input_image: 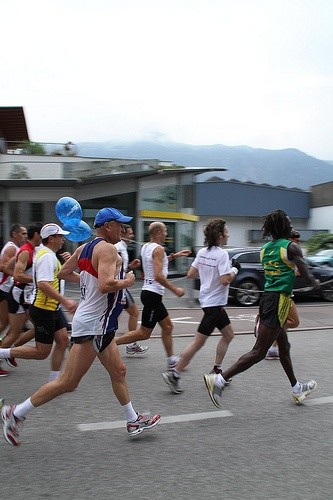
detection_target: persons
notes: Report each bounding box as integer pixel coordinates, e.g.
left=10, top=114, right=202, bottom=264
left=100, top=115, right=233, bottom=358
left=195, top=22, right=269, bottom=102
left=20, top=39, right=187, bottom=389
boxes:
left=113, top=221, right=149, bottom=356
left=162, top=218, right=241, bottom=394
left=203, top=212, right=320, bottom=408
left=0, top=223, right=72, bottom=378
left=0, top=223, right=79, bottom=393
left=254, top=229, right=302, bottom=359
left=113, top=221, right=192, bottom=369
left=0, top=207, right=162, bottom=448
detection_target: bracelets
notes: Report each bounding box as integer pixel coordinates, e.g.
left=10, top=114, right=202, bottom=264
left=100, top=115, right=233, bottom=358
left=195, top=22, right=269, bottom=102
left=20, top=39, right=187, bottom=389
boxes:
left=232, top=267, right=239, bottom=275
left=171, top=253, right=175, bottom=260
left=128, top=266, right=133, bottom=270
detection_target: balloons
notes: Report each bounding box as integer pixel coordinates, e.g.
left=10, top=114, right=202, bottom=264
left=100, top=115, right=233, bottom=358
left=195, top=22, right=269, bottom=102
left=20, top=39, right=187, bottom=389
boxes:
left=62, top=218, right=94, bottom=243
left=55, top=197, right=82, bottom=226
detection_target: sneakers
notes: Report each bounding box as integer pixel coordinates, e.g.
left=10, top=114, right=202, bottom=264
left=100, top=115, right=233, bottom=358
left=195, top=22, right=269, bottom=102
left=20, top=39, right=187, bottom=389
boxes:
left=162, top=370, right=183, bottom=394
left=127, top=412, right=161, bottom=437
left=168, top=358, right=178, bottom=370
left=0, top=370, right=10, bottom=377
left=292, top=379, right=317, bottom=403
left=5, top=357, right=17, bottom=367
left=265, top=347, right=281, bottom=360
left=1, top=404, right=26, bottom=446
left=204, top=373, right=224, bottom=408
left=126, top=342, right=149, bottom=356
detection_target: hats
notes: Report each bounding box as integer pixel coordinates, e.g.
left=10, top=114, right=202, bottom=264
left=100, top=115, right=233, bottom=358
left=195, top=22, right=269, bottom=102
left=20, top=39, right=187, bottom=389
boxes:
left=93, top=208, right=134, bottom=227
left=40, top=223, right=70, bottom=239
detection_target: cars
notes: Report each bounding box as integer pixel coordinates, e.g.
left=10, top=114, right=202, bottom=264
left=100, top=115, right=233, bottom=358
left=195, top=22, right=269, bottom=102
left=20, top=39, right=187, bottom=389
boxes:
left=193, top=246, right=333, bottom=307
